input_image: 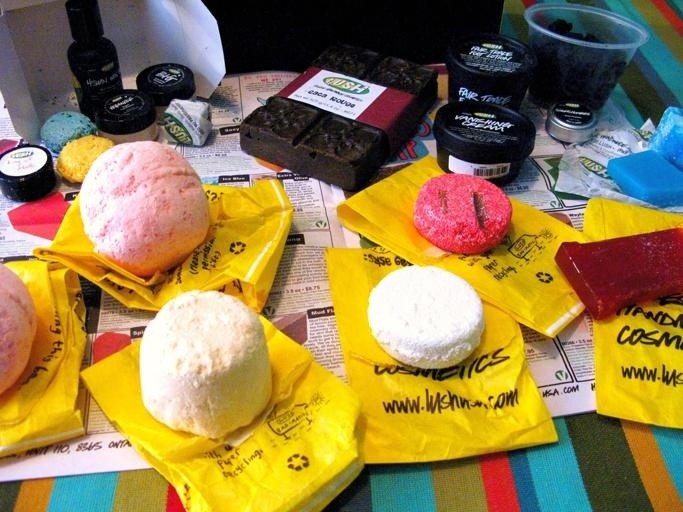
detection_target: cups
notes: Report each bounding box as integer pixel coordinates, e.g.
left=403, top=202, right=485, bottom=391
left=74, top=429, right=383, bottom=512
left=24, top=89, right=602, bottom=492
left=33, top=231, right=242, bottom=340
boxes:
left=523, top=2, right=652, bottom=112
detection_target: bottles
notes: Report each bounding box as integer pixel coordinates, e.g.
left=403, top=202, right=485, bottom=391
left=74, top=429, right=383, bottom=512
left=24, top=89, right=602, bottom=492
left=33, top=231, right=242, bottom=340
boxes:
left=66, top=1, right=123, bottom=114
